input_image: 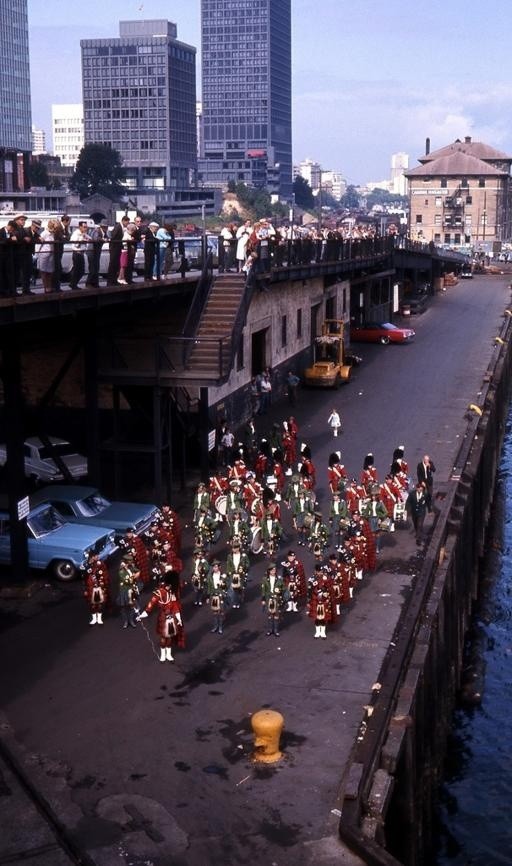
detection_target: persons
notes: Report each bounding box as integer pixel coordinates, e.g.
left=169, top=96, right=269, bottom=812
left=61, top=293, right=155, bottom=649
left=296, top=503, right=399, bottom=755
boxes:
left=218, top=219, right=399, bottom=274
left=190, top=442, right=435, bottom=637
left=82, top=505, right=184, bottom=666
left=1, top=209, right=177, bottom=298
left=404, top=227, right=511, bottom=265
left=327, top=409, right=342, bottom=437
left=247, top=368, right=303, bottom=462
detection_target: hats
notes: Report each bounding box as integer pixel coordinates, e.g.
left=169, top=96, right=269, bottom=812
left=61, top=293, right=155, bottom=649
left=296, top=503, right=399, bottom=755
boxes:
left=263, top=488, right=276, bottom=506
left=363, top=453, right=373, bottom=469
left=301, top=443, right=311, bottom=459
left=330, top=451, right=342, bottom=466
left=391, top=449, right=404, bottom=474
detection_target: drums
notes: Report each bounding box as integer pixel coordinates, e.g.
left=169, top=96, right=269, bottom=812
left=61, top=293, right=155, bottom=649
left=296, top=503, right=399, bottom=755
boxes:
left=249, top=496, right=259, bottom=514
left=393, top=502, right=408, bottom=522
left=380, top=518, right=392, bottom=532
left=215, top=494, right=227, bottom=515
left=249, top=525, right=264, bottom=555
left=303, top=514, right=315, bottom=529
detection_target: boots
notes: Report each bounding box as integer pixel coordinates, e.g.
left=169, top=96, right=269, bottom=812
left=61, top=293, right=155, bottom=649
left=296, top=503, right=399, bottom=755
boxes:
left=90, top=613, right=102, bottom=625
left=211, top=624, right=223, bottom=633
left=356, top=568, right=362, bottom=579
left=123, top=618, right=137, bottom=628
left=314, top=625, right=326, bottom=639
left=349, top=587, right=354, bottom=598
left=285, top=601, right=298, bottom=612
left=336, top=604, right=340, bottom=615
left=160, top=647, right=175, bottom=663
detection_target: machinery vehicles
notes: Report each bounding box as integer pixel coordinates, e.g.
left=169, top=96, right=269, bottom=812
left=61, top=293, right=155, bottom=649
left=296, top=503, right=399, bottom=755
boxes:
left=303, top=316, right=352, bottom=391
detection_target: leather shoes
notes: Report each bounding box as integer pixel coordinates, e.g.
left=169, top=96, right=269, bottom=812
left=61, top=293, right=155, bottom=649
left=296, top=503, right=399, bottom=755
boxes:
left=266, top=631, right=280, bottom=637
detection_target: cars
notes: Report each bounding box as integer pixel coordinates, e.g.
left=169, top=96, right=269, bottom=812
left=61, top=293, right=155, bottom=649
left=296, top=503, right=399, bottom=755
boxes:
left=3, top=434, right=163, bottom=579
left=346, top=321, right=416, bottom=348
left=1, top=210, right=223, bottom=277
